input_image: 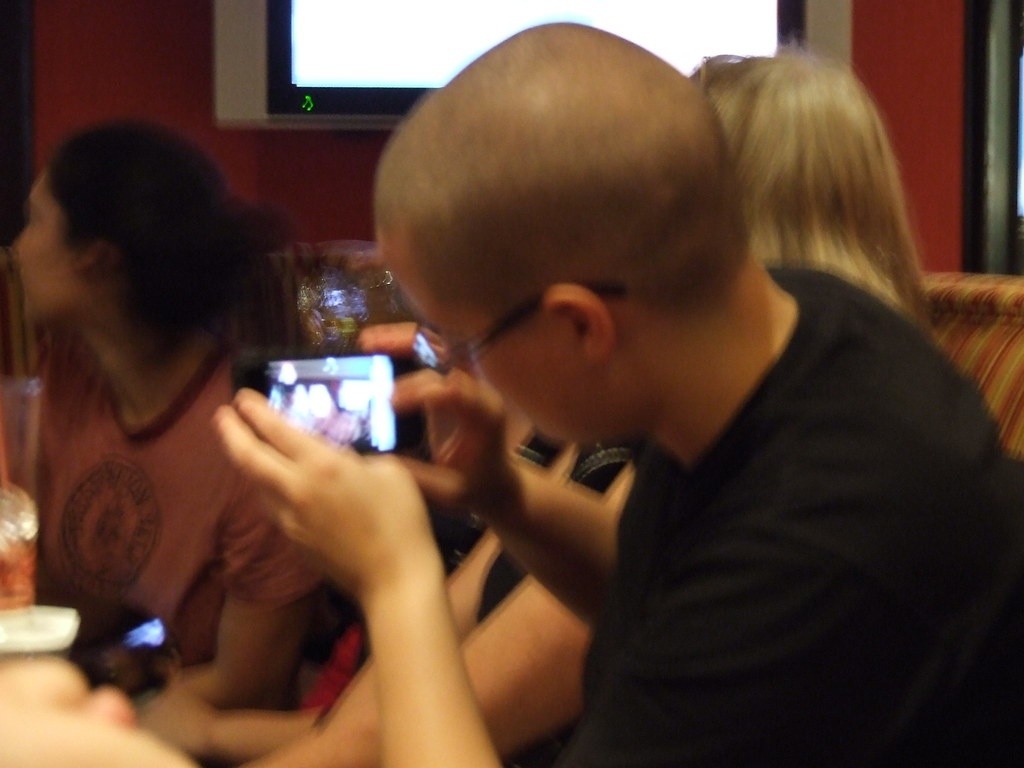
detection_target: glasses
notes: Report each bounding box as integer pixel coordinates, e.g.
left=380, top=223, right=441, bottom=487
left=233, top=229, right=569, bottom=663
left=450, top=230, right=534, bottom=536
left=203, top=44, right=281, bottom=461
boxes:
left=699, top=54, right=748, bottom=96
left=412, top=278, right=628, bottom=375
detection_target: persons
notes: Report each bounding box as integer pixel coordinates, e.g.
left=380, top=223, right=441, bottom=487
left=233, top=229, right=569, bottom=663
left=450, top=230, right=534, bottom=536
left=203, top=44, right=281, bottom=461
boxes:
left=214, top=21, right=1024, bottom=768
left=1, top=47, right=928, bottom=768
left=305, top=383, right=357, bottom=442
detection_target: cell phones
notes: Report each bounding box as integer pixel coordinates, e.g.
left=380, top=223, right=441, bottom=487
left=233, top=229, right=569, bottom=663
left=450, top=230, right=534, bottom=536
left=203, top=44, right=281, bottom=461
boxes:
left=235, top=345, right=425, bottom=460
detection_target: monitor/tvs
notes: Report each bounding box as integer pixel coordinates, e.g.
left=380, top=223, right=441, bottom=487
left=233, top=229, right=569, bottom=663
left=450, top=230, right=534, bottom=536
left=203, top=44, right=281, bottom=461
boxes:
left=211, top=0, right=853, bottom=133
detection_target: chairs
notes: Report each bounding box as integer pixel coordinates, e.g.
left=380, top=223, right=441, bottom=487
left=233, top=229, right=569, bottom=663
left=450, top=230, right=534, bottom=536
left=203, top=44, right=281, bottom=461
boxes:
left=922, top=272, right=1024, bottom=459
